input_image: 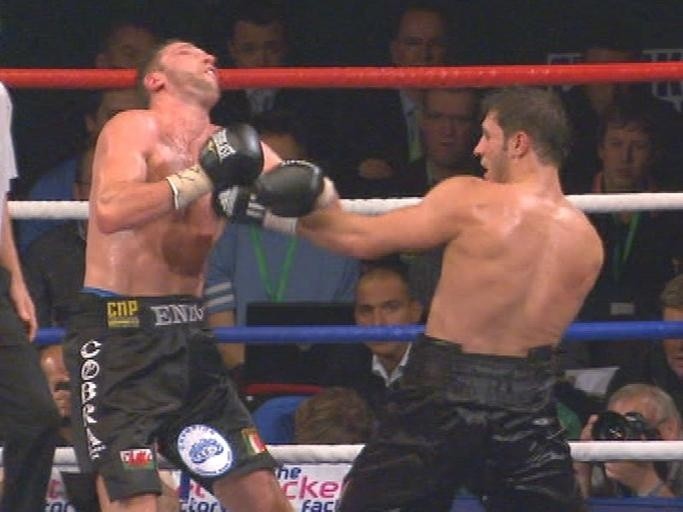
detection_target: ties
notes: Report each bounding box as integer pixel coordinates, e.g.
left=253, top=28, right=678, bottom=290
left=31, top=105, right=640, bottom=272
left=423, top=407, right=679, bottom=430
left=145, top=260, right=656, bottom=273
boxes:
left=408, top=105, right=422, bottom=166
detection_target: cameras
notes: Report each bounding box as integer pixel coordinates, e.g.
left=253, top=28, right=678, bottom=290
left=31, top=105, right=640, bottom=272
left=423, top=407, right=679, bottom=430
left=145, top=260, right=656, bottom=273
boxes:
left=592, top=411, right=668, bottom=477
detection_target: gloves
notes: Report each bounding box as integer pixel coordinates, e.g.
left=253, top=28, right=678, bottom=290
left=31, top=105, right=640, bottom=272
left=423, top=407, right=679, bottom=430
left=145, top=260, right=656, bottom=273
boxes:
left=199, top=123, right=263, bottom=190
left=212, top=184, right=270, bottom=226
left=257, top=159, right=323, bottom=218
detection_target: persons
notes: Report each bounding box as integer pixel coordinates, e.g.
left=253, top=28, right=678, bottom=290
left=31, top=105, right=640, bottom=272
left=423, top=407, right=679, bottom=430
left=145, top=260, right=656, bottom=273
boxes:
left=1, top=2, right=683, bottom=511
